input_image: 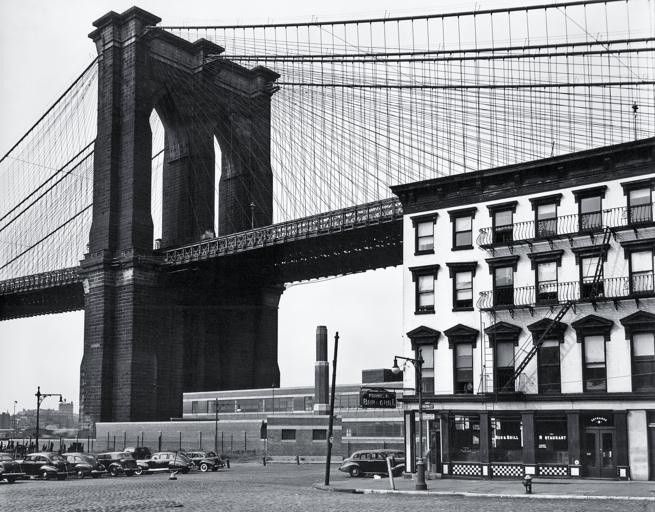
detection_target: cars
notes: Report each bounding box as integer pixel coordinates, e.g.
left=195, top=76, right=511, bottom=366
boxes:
left=0, top=447, right=225, bottom=484
left=338, top=449, right=406, bottom=478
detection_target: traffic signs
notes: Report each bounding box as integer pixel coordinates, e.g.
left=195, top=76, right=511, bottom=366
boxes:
left=359, top=391, right=396, bottom=408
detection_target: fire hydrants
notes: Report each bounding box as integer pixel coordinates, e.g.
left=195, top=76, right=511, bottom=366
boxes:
left=522, top=475, right=532, bottom=494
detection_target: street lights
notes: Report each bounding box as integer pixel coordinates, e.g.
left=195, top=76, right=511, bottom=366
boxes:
left=391, top=350, right=427, bottom=490
left=213, top=396, right=242, bottom=452
left=35, top=386, right=63, bottom=452
left=249, top=202, right=256, bottom=228
left=631, top=101, right=638, bottom=141
